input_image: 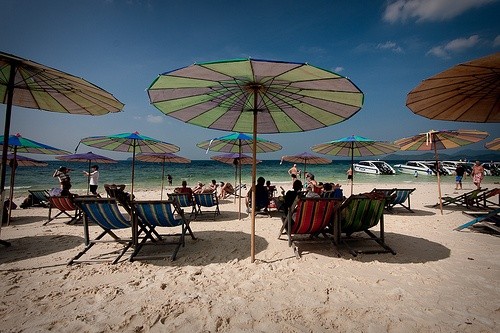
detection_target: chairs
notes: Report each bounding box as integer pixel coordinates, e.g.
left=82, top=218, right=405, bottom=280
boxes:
left=75, top=194, right=102, bottom=223
left=167, top=192, right=198, bottom=220
left=322, top=193, right=398, bottom=257
left=128, top=198, right=198, bottom=262
left=194, top=193, right=221, bottom=221
left=369, top=188, right=396, bottom=214
left=434, top=187, right=488, bottom=209
left=42, top=196, right=83, bottom=225
left=264, top=185, right=277, bottom=200
left=277, top=196, right=346, bottom=259
left=66, top=197, right=146, bottom=265
left=385, top=188, right=416, bottom=214
left=453, top=209, right=500, bottom=233
left=247, top=192, right=271, bottom=219
left=107, top=191, right=135, bottom=215
left=29, top=190, right=56, bottom=208
left=462, top=188, right=500, bottom=209
left=224, top=184, right=246, bottom=200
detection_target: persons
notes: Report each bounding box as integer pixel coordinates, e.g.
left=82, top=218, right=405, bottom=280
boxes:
left=414, top=170, right=418, bottom=178
left=219, top=181, right=234, bottom=198
left=470, top=160, right=484, bottom=189
left=200, top=179, right=217, bottom=193
left=266, top=181, right=270, bottom=186
left=287, top=163, right=299, bottom=189
left=346, top=167, right=353, bottom=184
left=106, top=183, right=126, bottom=191
left=453, top=163, right=464, bottom=189
left=489, top=160, right=500, bottom=175
left=458, top=156, right=469, bottom=162
left=308, top=185, right=343, bottom=198
left=320, top=182, right=324, bottom=185
left=280, top=180, right=304, bottom=215
left=190, top=181, right=202, bottom=195
left=429, top=159, right=445, bottom=173
left=83, top=165, right=100, bottom=195
left=166, top=173, right=173, bottom=185
left=245, top=177, right=270, bottom=213
left=173, top=179, right=193, bottom=194
left=52, top=166, right=73, bottom=193
left=306, top=172, right=317, bottom=187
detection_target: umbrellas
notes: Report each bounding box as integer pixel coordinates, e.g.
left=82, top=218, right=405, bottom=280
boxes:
left=0, top=134, right=72, bottom=227
left=405, top=53, right=500, bottom=123
left=196, top=132, right=282, bottom=219
left=394, top=128, right=489, bottom=215
left=309, top=134, right=399, bottom=196
left=135, top=151, right=191, bottom=201
left=144, top=56, right=364, bottom=264
left=1, top=153, right=49, bottom=198
left=210, top=152, right=262, bottom=204
left=483, top=137, right=500, bottom=151
left=0, top=51, right=125, bottom=192
left=280, top=151, right=331, bottom=183
left=80, top=131, right=180, bottom=201
left=56, top=152, right=117, bottom=195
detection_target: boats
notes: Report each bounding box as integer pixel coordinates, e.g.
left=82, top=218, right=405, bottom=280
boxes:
left=443, top=160, right=476, bottom=175
left=479, top=161, right=500, bottom=175
left=353, top=160, right=397, bottom=174
left=394, top=160, right=450, bottom=175
